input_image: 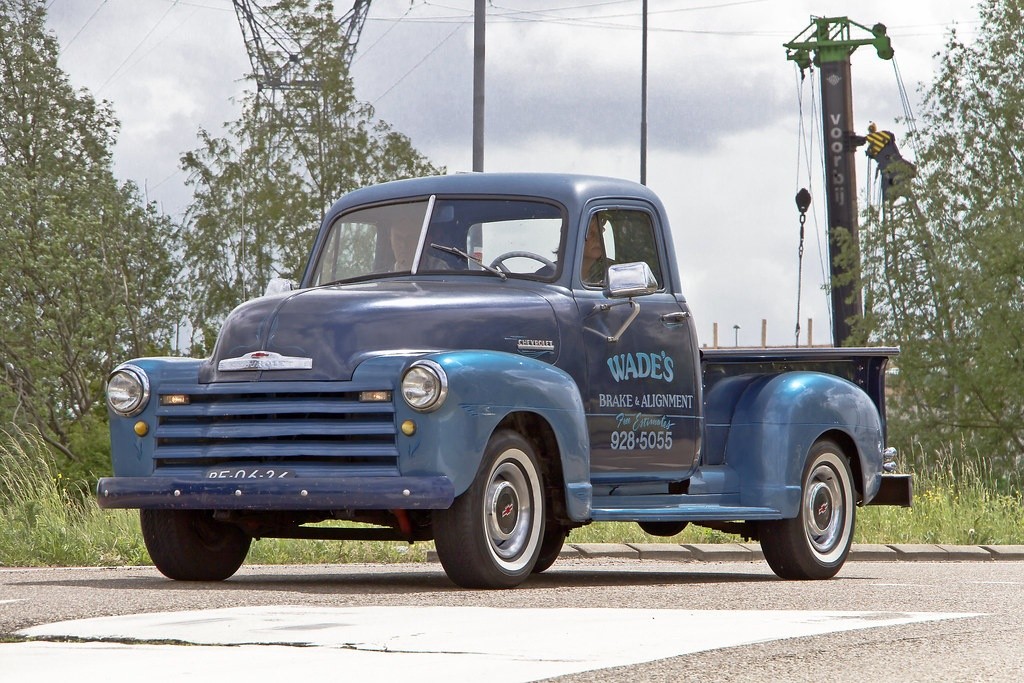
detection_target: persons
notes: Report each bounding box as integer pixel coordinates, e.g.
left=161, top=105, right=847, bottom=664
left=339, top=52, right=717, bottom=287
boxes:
left=530, top=207, right=625, bottom=289
left=371, top=207, right=453, bottom=282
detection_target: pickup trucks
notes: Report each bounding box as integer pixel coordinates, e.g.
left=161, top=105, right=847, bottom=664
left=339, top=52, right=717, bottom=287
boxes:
left=96, top=172, right=916, bottom=589
